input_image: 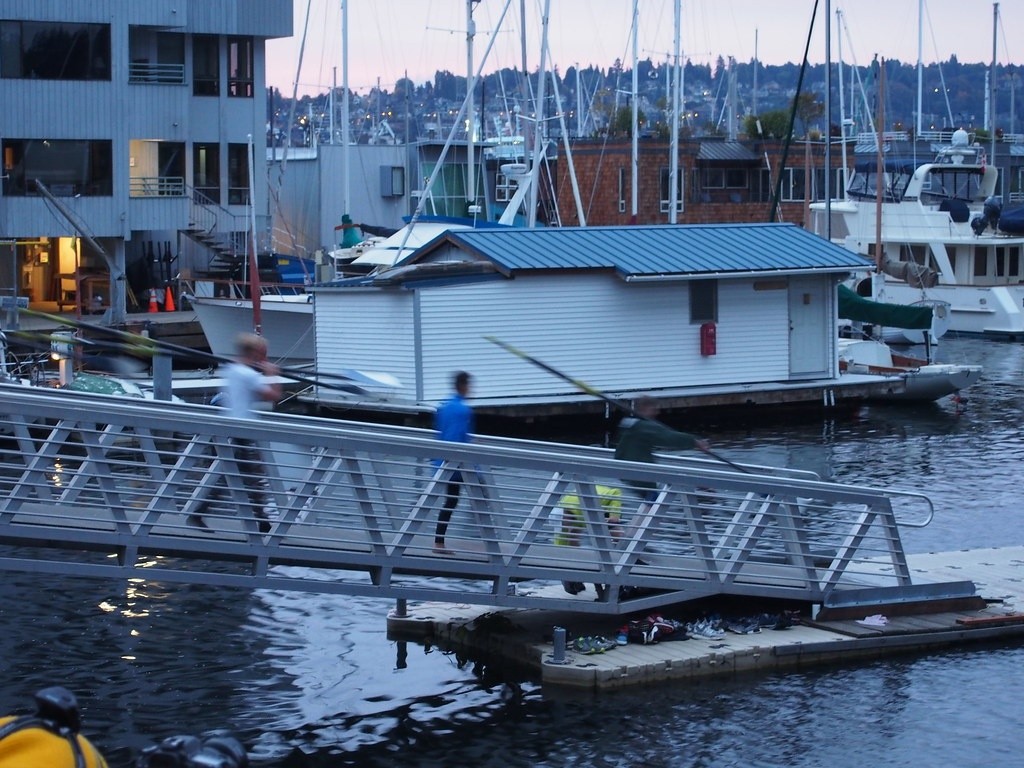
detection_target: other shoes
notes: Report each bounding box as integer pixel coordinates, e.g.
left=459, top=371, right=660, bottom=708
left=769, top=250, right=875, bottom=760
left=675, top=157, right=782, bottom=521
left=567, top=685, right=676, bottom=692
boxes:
left=855, top=614, right=888, bottom=626
left=186, top=516, right=215, bottom=533
left=543, top=632, right=574, bottom=649
left=258, top=523, right=271, bottom=532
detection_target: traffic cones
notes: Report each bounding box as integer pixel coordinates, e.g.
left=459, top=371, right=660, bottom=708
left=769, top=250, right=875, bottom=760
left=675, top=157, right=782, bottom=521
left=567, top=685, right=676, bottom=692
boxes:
left=165, top=285, right=178, bottom=313
left=148, top=290, right=161, bottom=312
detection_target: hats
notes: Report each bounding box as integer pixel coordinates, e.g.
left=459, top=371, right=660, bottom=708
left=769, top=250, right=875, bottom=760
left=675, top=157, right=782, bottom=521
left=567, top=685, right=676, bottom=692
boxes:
left=549, top=507, right=564, bottom=532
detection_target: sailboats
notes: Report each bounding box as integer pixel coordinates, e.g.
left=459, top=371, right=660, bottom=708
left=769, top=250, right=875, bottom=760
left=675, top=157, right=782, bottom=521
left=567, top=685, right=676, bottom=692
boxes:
left=186, top=134, right=314, bottom=377
left=772, top=0, right=1024, bottom=402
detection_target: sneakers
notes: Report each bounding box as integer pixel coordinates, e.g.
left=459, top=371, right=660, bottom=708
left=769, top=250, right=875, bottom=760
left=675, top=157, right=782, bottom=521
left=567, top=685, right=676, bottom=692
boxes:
left=593, top=635, right=616, bottom=650
left=572, top=637, right=595, bottom=654
left=616, top=611, right=799, bottom=646
left=585, top=636, right=605, bottom=654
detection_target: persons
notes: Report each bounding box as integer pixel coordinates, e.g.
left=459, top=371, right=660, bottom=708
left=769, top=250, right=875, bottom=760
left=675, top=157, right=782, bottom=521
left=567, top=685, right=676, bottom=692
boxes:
left=549, top=396, right=710, bottom=546
left=423, top=372, right=489, bottom=557
left=189, top=332, right=278, bottom=533
